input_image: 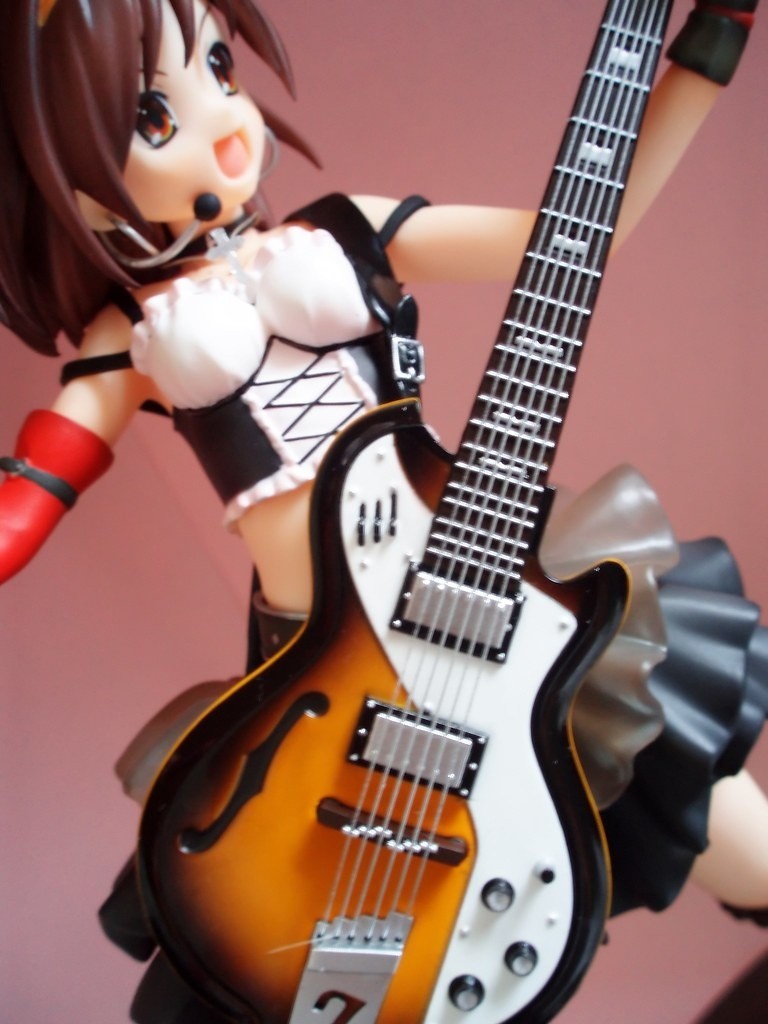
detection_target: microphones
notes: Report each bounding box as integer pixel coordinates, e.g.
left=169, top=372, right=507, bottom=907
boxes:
left=95, top=192, right=221, bottom=269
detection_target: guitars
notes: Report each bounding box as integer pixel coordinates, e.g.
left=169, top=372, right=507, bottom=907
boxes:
left=136, top=0, right=675, bottom=1024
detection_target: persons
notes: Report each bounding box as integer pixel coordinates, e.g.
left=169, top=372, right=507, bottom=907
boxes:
left=0, top=0, right=768, bottom=1024
